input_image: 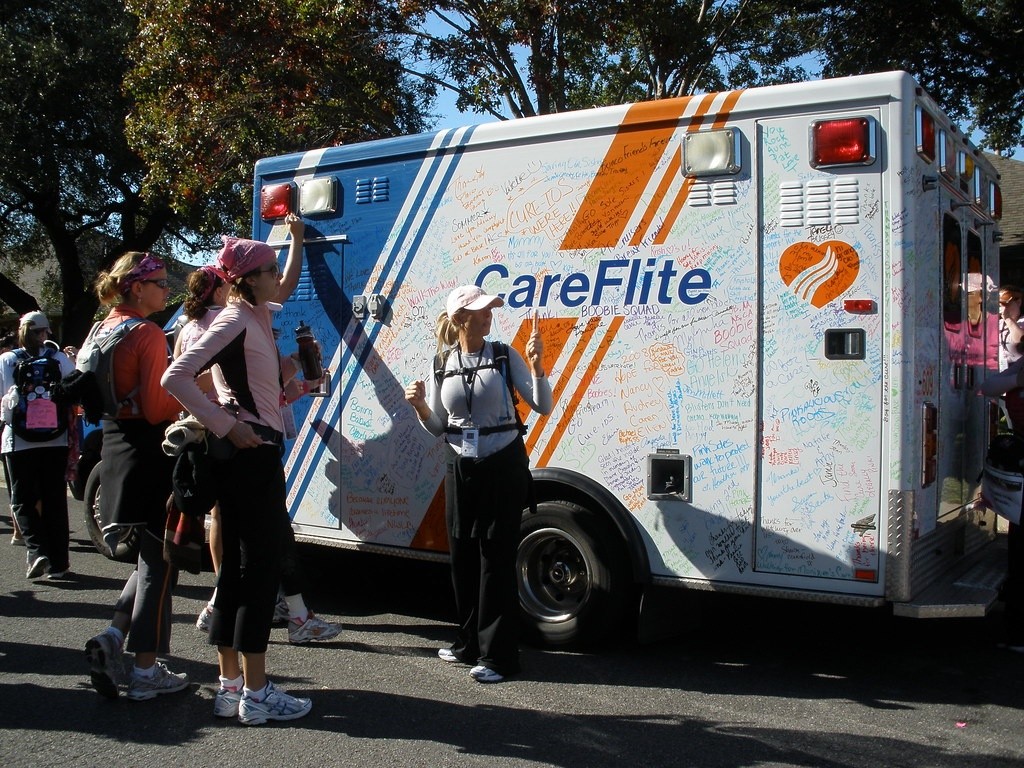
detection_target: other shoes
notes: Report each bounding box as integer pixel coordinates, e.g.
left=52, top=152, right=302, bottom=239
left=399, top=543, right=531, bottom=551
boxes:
left=11, top=536, right=26, bottom=545
left=996, top=630, right=1024, bottom=652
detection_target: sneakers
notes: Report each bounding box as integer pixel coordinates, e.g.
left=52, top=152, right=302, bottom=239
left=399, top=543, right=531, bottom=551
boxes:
left=288, top=610, right=341, bottom=643
left=214, top=676, right=245, bottom=717
left=25, top=551, right=48, bottom=580
left=437, top=648, right=466, bottom=663
left=270, top=595, right=290, bottom=622
left=238, top=679, right=312, bottom=726
left=126, top=662, right=189, bottom=701
left=47, top=569, right=80, bottom=583
left=469, top=665, right=504, bottom=682
left=195, top=607, right=212, bottom=633
left=85, top=630, right=123, bottom=698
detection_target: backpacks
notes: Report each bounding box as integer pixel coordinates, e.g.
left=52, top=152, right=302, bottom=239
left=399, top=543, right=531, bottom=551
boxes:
left=980, top=434, right=1023, bottom=526
left=9, top=349, right=71, bottom=443
left=75, top=319, right=153, bottom=427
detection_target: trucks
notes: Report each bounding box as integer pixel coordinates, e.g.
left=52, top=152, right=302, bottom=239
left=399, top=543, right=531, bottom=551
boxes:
left=65, top=68, right=1024, bottom=661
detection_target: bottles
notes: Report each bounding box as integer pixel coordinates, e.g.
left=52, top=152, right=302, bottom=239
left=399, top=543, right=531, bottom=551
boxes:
left=295, top=320, right=322, bottom=380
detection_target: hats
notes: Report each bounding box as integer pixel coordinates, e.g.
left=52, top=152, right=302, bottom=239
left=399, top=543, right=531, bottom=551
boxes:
left=20, top=310, right=52, bottom=334
left=446, top=285, right=504, bottom=321
left=998, top=284, right=1022, bottom=303
left=959, top=272, right=997, bottom=293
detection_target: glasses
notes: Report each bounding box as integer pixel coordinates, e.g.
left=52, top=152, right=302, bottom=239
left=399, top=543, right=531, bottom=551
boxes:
left=126, top=279, right=169, bottom=289
left=244, top=262, right=281, bottom=279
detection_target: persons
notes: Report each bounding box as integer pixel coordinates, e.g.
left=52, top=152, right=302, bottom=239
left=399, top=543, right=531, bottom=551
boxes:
left=75, top=252, right=188, bottom=700
left=0, top=311, right=79, bottom=579
left=160, top=213, right=342, bottom=726
left=997, top=281, right=1024, bottom=463
left=949, top=272, right=997, bottom=401
left=405, top=285, right=552, bottom=684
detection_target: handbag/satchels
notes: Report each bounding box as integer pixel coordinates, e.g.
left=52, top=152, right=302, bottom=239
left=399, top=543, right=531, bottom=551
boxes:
left=171, top=430, right=229, bottom=517
